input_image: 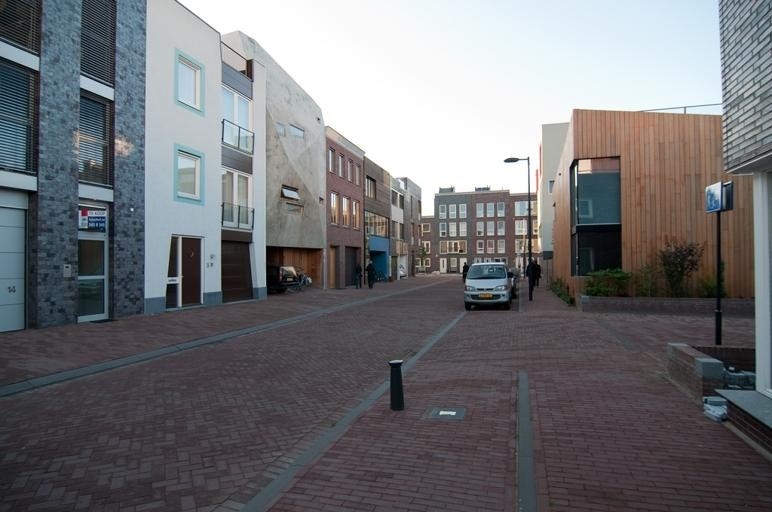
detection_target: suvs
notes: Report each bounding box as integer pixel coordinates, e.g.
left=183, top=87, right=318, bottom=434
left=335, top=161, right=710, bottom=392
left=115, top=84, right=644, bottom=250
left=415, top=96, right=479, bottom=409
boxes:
left=267, top=265, right=300, bottom=294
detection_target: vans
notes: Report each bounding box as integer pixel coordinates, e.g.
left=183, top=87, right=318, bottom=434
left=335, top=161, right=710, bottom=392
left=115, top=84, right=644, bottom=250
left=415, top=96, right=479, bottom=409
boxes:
left=463, top=261, right=515, bottom=310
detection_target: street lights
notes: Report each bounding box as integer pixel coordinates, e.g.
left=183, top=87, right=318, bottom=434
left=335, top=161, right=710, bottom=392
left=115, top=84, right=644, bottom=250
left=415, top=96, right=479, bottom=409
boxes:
left=503, top=158, right=531, bottom=268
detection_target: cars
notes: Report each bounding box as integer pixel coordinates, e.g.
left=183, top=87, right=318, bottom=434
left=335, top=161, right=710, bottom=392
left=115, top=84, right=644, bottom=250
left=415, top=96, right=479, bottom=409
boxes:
left=399, top=264, right=408, bottom=277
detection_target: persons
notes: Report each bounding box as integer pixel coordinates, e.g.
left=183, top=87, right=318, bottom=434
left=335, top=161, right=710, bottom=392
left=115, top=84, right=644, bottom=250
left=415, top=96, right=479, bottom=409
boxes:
left=534, top=260, right=541, bottom=286
left=463, top=262, right=469, bottom=282
left=525, top=262, right=537, bottom=301
left=354, top=261, right=365, bottom=291
left=364, top=259, right=376, bottom=288
